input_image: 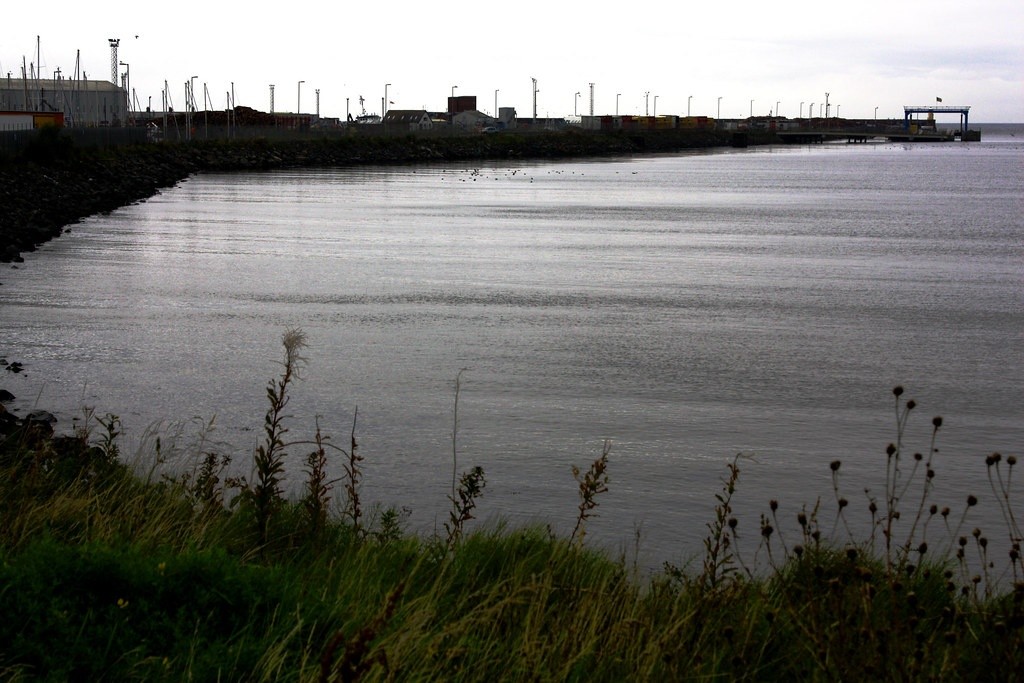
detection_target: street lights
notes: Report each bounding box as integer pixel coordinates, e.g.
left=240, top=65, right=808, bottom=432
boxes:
left=532, top=77, right=539, bottom=124
left=190, top=76, right=199, bottom=128
left=588, top=82, right=595, bottom=116
left=347, top=98, right=349, bottom=117
left=269, top=85, right=275, bottom=116
left=315, top=89, right=320, bottom=115
left=298, top=81, right=305, bottom=115
left=616, top=93, right=622, bottom=115
left=750, top=99, right=755, bottom=117
left=717, top=97, right=722, bottom=125
left=688, top=95, right=693, bottom=116
left=119, top=61, right=130, bottom=111
left=385, top=84, right=391, bottom=114
left=494, top=89, right=500, bottom=118
left=52, top=71, right=61, bottom=110
left=574, top=91, right=580, bottom=117
left=775, top=102, right=782, bottom=117
left=798, top=92, right=841, bottom=128
left=452, top=86, right=457, bottom=117
left=654, top=95, right=659, bottom=117
left=107, top=38, right=120, bottom=119
left=874, top=106, right=879, bottom=120
